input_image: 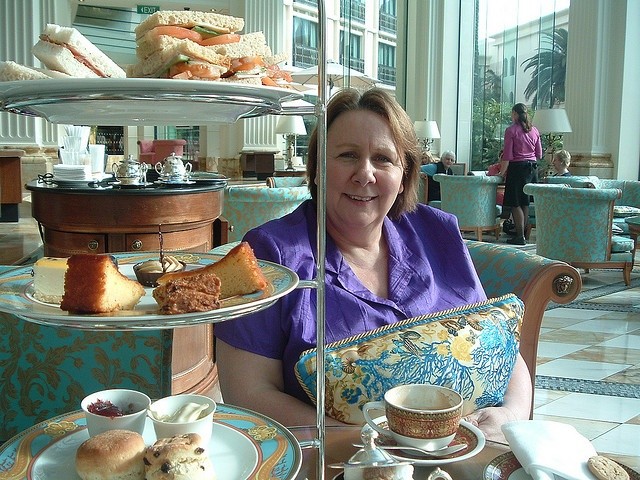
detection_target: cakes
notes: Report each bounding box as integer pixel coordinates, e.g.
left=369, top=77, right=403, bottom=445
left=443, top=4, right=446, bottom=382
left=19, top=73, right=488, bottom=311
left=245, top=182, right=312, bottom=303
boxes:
left=33, top=257, right=70, bottom=305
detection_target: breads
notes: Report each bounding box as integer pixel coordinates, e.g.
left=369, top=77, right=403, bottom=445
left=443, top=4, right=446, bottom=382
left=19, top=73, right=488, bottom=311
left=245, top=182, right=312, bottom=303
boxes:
left=0, top=60, right=52, bottom=82
left=152, top=273, right=221, bottom=315
left=156, top=241, right=269, bottom=300
left=22, top=65, right=73, bottom=78
left=144, top=433, right=209, bottom=480
left=60, top=252, right=146, bottom=314
left=75, top=429, right=144, bottom=480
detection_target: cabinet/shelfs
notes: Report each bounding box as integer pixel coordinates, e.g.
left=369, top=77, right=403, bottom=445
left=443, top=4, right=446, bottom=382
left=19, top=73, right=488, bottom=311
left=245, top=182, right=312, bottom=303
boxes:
left=239, top=150, right=256, bottom=178
left=0, top=149, right=26, bottom=222
left=254, top=150, right=280, bottom=181
left=274, top=169, right=309, bottom=176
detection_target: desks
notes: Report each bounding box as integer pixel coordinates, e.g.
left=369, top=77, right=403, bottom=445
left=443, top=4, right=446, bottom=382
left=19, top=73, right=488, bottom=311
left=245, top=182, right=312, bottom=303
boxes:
left=625, top=217, right=640, bottom=271
left=24, top=169, right=235, bottom=398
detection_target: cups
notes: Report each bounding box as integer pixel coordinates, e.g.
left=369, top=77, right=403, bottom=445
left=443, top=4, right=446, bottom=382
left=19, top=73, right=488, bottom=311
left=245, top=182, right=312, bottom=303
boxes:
left=81, top=389, right=152, bottom=438
left=362, top=384, right=463, bottom=454
left=147, top=394, right=217, bottom=444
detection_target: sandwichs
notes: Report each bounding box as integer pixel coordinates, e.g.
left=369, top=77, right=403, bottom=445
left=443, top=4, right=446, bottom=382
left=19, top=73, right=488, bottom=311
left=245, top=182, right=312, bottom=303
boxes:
left=31, top=23, right=126, bottom=78
left=126, top=10, right=294, bottom=89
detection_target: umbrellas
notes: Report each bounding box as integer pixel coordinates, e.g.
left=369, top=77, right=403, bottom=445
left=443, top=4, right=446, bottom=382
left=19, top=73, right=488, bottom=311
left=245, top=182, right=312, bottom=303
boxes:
left=289, top=59, right=381, bottom=99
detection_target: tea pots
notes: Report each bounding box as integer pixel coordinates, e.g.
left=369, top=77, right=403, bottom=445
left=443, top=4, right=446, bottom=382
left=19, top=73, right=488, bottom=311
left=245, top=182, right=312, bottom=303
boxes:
left=112, top=155, right=148, bottom=184
left=154, top=152, right=193, bottom=181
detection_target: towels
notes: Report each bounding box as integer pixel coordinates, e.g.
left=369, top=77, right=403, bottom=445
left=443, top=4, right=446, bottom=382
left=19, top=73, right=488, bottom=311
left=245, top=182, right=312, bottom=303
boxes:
left=501, top=422, right=599, bottom=480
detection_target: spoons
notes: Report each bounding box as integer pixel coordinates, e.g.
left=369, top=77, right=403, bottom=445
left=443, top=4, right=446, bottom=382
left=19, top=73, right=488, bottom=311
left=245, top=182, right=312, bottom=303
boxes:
left=351, top=441, right=468, bottom=458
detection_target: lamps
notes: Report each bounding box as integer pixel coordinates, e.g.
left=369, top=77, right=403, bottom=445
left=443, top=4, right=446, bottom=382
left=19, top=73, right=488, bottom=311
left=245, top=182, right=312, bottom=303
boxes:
left=532, top=108, right=574, bottom=176
left=412, top=119, right=442, bottom=151
left=274, top=114, right=308, bottom=170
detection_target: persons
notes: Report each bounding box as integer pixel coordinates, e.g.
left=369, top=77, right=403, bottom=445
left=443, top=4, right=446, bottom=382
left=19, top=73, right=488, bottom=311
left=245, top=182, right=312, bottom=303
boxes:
left=420, top=151, right=434, bottom=164
left=498, top=103, right=542, bottom=245
left=552, top=150, right=572, bottom=176
left=437, top=151, right=455, bottom=175
left=487, top=150, right=507, bottom=206
left=213, top=86, right=532, bottom=446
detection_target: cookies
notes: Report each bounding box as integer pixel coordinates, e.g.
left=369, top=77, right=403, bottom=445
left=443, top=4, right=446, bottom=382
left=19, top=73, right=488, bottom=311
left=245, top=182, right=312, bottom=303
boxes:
left=588, top=455, right=630, bottom=480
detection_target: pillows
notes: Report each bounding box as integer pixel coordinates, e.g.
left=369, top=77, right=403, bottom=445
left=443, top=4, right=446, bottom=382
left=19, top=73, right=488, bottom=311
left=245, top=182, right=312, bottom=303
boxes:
left=613, top=206, right=640, bottom=216
left=294, top=293, right=524, bottom=427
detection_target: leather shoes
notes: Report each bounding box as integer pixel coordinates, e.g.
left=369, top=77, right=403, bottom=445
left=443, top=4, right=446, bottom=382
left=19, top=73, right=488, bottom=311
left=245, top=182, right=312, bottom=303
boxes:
left=506, top=236, right=526, bottom=245
left=508, top=230, right=527, bottom=236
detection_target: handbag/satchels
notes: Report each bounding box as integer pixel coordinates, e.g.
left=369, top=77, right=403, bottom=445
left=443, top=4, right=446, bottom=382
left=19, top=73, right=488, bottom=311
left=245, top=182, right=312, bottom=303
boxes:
left=503, top=218, right=515, bottom=235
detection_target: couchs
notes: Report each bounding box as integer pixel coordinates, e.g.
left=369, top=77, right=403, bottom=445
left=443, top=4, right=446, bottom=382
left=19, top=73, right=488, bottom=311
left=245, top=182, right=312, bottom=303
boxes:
left=210, top=238, right=581, bottom=421
left=574, top=179, right=640, bottom=234
left=525, top=176, right=598, bottom=240
left=220, top=183, right=312, bottom=243
left=524, top=182, right=635, bottom=288
left=137, top=139, right=187, bottom=166
left=417, top=162, right=466, bottom=209
left=1, top=263, right=171, bottom=444
left=1, top=146, right=61, bottom=187
left=433, top=174, right=503, bottom=243
left=265, top=176, right=309, bottom=188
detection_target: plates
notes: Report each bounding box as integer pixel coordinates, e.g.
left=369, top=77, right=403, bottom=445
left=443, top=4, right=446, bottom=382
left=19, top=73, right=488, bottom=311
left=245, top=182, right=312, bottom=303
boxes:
left=483, top=450, right=640, bottom=480
left=108, top=181, right=153, bottom=189
left=0, top=252, right=300, bottom=330
left=154, top=181, right=196, bottom=185
left=1, top=78, right=303, bottom=126
left=52, top=179, right=95, bottom=188
left=0, top=402, right=303, bottom=480
left=361, top=414, right=486, bottom=468
